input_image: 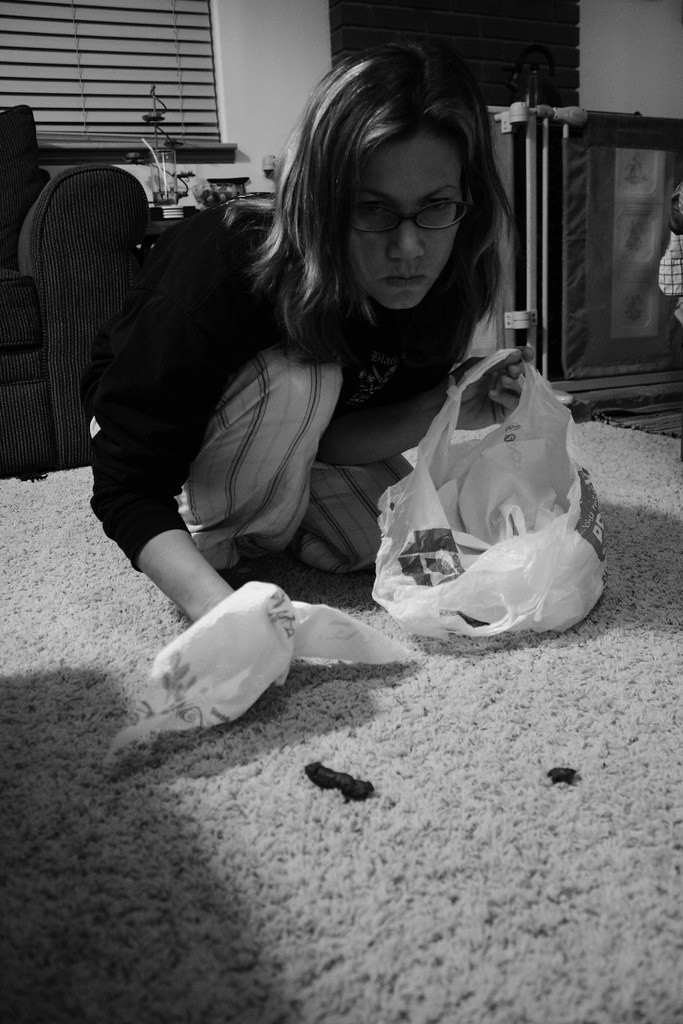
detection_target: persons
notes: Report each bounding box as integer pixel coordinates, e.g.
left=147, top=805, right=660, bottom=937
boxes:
left=659, top=183, right=683, bottom=326
left=91, top=42, right=537, bottom=625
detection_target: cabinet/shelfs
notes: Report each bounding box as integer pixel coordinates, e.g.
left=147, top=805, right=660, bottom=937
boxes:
left=502, top=113, right=683, bottom=394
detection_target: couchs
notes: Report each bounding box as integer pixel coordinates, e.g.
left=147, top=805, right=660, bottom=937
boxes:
left=0, top=169, right=165, bottom=481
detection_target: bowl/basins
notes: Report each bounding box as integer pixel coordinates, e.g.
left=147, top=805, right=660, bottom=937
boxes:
left=192, top=182, right=236, bottom=208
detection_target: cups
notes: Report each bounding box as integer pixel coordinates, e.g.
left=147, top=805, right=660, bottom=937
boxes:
left=261, top=154, right=276, bottom=176
left=149, top=149, right=177, bottom=204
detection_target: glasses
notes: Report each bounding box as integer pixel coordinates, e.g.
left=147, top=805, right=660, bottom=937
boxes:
left=350, top=182, right=473, bottom=233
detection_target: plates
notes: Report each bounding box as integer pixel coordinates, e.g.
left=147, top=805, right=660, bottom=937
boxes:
left=206, top=176, right=250, bottom=185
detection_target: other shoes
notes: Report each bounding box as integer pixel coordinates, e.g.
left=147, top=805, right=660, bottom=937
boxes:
left=218, top=560, right=272, bottom=591
left=266, top=539, right=301, bottom=572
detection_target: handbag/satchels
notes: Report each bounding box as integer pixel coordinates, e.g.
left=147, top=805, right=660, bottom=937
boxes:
left=372, top=347, right=608, bottom=639
